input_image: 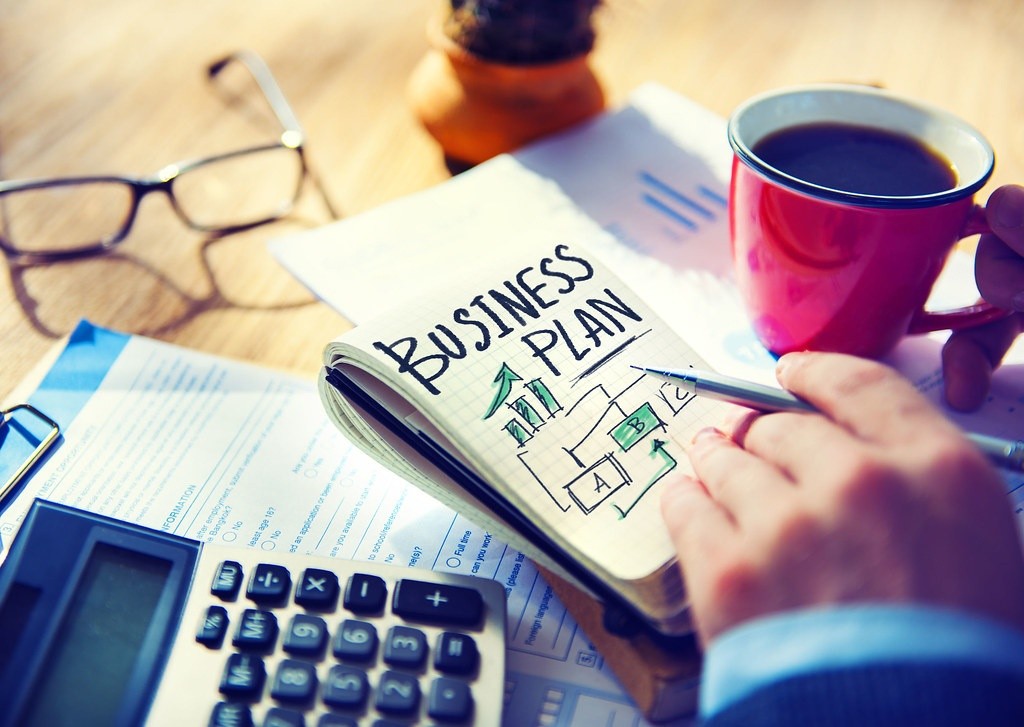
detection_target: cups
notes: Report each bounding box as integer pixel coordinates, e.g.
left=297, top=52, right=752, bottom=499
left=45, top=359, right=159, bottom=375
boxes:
left=726, top=80, right=1014, bottom=357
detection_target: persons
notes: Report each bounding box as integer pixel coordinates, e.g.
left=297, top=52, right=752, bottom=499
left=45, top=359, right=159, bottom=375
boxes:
left=663, top=185, right=1024, bottom=727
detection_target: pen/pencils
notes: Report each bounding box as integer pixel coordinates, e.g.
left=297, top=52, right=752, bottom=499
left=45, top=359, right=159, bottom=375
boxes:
left=630, top=361, right=1024, bottom=476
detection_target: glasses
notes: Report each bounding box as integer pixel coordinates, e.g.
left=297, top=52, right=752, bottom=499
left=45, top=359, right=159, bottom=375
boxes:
left=1, top=46, right=307, bottom=263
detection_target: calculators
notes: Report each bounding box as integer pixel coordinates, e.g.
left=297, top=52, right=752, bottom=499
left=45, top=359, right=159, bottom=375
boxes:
left=0, top=495, right=507, bottom=727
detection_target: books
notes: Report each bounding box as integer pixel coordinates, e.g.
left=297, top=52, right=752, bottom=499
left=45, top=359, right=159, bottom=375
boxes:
left=318, top=223, right=769, bottom=724
